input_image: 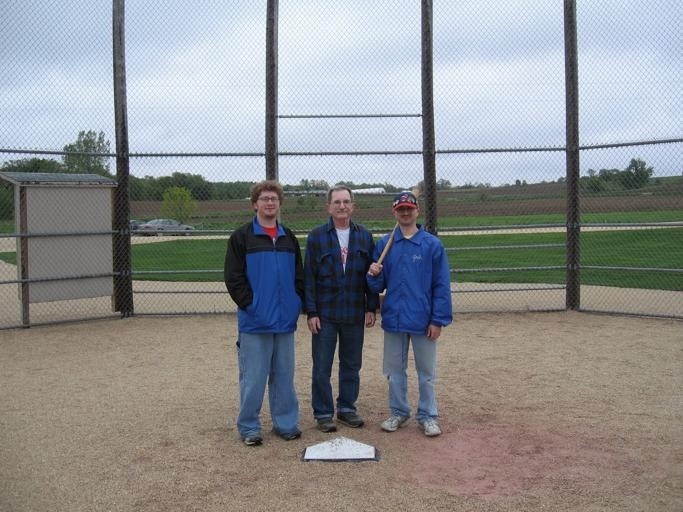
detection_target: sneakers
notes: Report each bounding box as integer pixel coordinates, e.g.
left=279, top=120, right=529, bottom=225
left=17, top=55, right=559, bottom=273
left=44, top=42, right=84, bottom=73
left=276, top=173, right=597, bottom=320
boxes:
left=242, top=435, right=262, bottom=446
left=274, top=427, right=302, bottom=440
left=335, top=410, right=365, bottom=428
left=380, top=414, right=412, bottom=432
left=417, top=417, right=442, bottom=436
left=314, top=417, right=338, bottom=433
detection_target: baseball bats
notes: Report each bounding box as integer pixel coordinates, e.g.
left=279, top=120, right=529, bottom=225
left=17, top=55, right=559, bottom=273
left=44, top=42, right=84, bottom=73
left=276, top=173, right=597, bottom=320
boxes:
left=377, top=187, right=419, bottom=264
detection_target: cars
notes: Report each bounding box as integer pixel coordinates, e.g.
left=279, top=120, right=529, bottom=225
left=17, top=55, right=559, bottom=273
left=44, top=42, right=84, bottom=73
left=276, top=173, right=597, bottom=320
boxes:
left=130, top=219, right=195, bottom=236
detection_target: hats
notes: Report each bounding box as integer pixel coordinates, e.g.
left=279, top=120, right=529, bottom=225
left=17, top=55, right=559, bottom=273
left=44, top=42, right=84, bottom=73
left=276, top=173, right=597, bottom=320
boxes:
left=392, top=190, right=419, bottom=210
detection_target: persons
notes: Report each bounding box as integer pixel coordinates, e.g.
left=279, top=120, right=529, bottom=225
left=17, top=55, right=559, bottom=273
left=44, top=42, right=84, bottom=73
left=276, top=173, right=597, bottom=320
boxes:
left=224, top=180, right=304, bottom=447
left=365, top=192, right=453, bottom=436
left=303, top=187, right=380, bottom=433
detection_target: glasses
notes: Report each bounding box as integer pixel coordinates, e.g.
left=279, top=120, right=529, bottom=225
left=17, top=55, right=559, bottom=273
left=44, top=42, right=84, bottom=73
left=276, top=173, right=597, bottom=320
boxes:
left=257, top=196, right=280, bottom=202
